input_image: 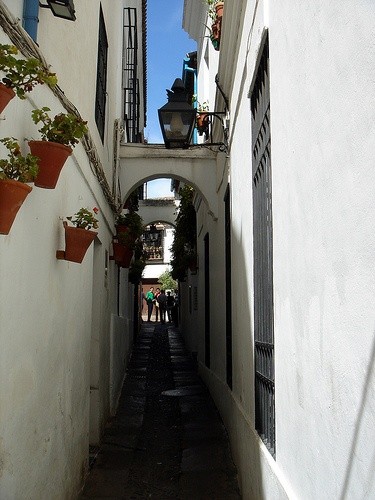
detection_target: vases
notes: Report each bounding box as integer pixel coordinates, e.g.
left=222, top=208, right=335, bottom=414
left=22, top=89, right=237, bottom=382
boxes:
left=62, top=222, right=98, bottom=265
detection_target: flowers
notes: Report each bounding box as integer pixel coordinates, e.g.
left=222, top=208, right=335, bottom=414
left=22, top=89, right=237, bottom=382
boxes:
left=67, top=206, right=100, bottom=233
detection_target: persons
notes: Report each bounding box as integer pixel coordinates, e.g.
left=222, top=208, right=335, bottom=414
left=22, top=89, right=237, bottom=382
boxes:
left=173, top=289, right=178, bottom=307
left=154, top=288, right=161, bottom=323
left=166, top=292, right=175, bottom=323
left=146, top=287, right=155, bottom=322
left=158, top=290, right=167, bottom=324
left=142, top=287, right=146, bottom=309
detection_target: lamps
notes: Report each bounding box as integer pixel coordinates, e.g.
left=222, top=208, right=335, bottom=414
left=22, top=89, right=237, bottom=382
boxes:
left=147, top=223, right=161, bottom=242
left=158, top=77, right=230, bottom=159
left=39, top=0, right=77, bottom=21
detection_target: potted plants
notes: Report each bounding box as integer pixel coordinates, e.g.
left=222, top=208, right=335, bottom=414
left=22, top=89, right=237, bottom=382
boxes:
left=0, top=44, right=59, bottom=114
left=112, top=212, right=145, bottom=270
left=0, top=135, right=42, bottom=235
left=28, top=106, right=90, bottom=190
left=192, top=93, right=210, bottom=130
left=201, top=0, right=224, bottom=51
